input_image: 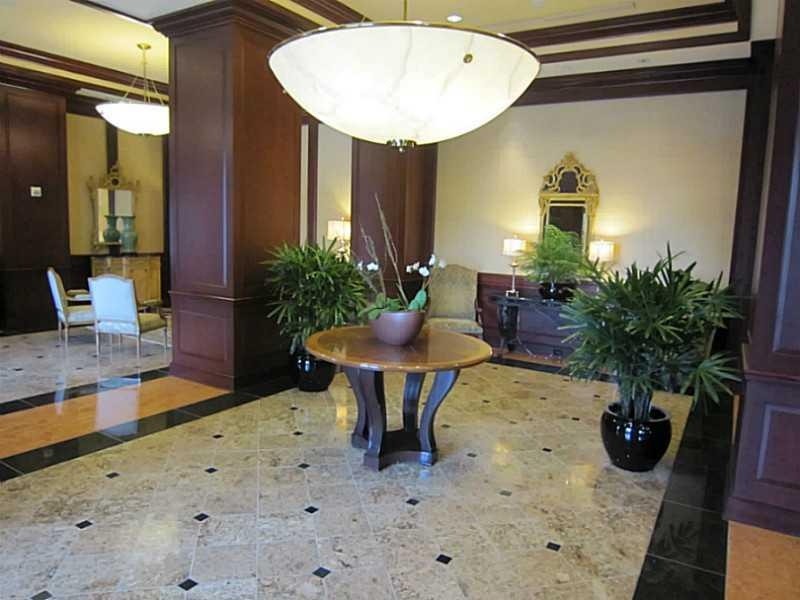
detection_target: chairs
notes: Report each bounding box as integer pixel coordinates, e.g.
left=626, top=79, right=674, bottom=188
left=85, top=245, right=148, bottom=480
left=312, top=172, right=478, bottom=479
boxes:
left=88, top=274, right=168, bottom=360
left=47, top=267, right=95, bottom=350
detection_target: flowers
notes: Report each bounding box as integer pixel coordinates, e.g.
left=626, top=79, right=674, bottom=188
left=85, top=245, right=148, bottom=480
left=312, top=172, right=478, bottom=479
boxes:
left=356, top=256, right=448, bottom=320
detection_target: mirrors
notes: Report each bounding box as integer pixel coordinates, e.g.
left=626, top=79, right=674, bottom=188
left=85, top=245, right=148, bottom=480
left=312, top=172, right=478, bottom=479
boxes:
left=300, top=107, right=352, bottom=250
left=86, top=162, right=140, bottom=252
left=539, top=153, right=599, bottom=259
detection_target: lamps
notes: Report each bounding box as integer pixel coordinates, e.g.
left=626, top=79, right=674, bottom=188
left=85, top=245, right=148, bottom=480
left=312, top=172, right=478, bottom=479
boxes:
left=327, top=218, right=351, bottom=263
left=95, top=43, right=170, bottom=137
left=588, top=240, right=615, bottom=293
left=502, top=234, right=527, bottom=298
left=266, top=0, right=542, bottom=157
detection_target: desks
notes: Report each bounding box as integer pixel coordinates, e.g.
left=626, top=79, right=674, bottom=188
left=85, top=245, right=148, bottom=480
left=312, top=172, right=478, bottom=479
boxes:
left=303, top=329, right=493, bottom=472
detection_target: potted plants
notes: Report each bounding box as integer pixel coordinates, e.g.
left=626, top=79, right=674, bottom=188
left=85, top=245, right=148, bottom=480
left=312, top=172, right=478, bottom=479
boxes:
left=558, top=240, right=759, bottom=473
left=513, top=224, right=587, bottom=302
left=257, top=234, right=372, bottom=391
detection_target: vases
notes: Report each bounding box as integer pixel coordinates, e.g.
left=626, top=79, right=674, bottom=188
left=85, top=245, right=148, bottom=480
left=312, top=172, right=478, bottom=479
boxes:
left=102, top=215, right=121, bottom=242
left=121, top=216, right=138, bottom=252
left=368, top=310, right=426, bottom=346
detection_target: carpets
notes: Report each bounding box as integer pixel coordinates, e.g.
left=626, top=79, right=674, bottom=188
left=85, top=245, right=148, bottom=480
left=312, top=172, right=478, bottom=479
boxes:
left=86, top=326, right=172, bottom=348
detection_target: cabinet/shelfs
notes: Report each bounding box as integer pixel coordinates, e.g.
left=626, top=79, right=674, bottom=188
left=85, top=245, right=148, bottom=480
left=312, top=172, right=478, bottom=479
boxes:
left=71, top=253, right=165, bottom=313
